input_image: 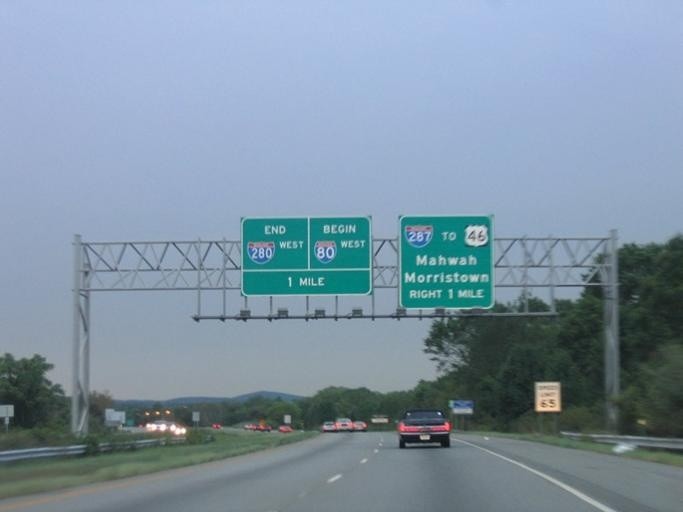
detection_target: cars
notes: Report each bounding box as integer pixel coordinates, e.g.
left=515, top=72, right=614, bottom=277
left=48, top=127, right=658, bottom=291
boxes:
left=321, top=418, right=367, bottom=433
left=144, top=419, right=187, bottom=436
left=394, top=409, right=451, bottom=448
left=211, top=423, right=220, bottom=429
left=242, top=423, right=292, bottom=433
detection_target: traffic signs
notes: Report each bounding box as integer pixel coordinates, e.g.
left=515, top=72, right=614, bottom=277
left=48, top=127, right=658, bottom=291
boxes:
left=397, top=214, right=495, bottom=309
left=239, top=214, right=372, bottom=296
left=533, top=381, right=561, bottom=413
